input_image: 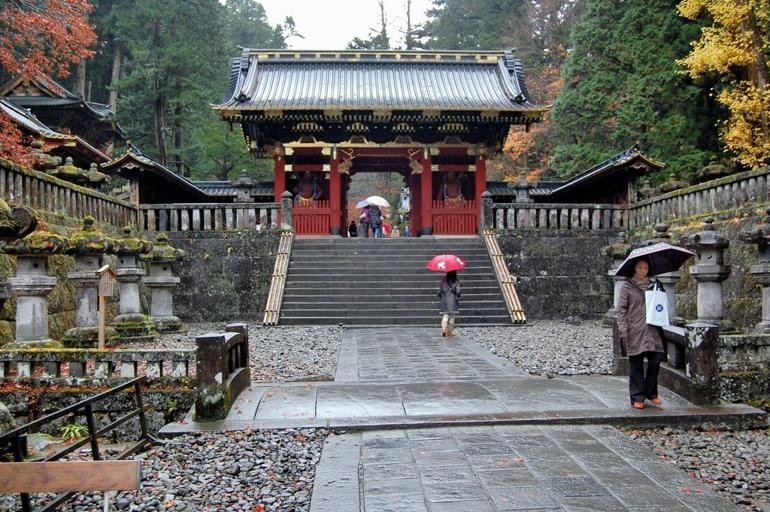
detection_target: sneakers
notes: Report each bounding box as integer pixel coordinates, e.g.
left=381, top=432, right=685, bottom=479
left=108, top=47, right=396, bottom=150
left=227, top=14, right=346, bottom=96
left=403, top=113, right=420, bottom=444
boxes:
left=631, top=398, right=660, bottom=409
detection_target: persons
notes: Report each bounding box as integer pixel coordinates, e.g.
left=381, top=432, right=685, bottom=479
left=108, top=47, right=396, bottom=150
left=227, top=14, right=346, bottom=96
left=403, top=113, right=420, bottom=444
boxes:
left=349, top=220, right=358, bottom=238
left=359, top=205, right=371, bottom=238
left=367, top=205, right=382, bottom=239
left=381, top=220, right=410, bottom=238
left=618, top=256, right=667, bottom=409
left=437, top=270, right=462, bottom=337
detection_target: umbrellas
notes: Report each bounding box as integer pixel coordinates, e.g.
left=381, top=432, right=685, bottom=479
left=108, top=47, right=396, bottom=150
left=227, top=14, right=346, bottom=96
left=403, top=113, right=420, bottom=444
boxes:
left=364, top=196, right=390, bottom=207
left=614, top=242, right=697, bottom=293
left=355, top=200, right=369, bottom=209
left=425, top=252, right=466, bottom=272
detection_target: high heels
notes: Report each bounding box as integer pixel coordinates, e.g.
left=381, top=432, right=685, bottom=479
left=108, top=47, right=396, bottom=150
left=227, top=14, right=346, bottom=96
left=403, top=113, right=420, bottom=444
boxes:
left=443, top=331, right=453, bottom=337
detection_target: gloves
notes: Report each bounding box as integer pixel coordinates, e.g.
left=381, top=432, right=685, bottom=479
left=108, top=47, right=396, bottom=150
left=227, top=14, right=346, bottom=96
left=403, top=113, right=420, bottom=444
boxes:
left=654, top=279, right=664, bottom=292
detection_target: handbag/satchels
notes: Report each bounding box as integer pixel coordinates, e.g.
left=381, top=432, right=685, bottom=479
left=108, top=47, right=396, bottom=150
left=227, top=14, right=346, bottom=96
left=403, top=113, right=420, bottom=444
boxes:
left=376, top=216, right=382, bottom=225
left=359, top=212, right=366, bottom=222
left=645, top=290, right=674, bottom=327
